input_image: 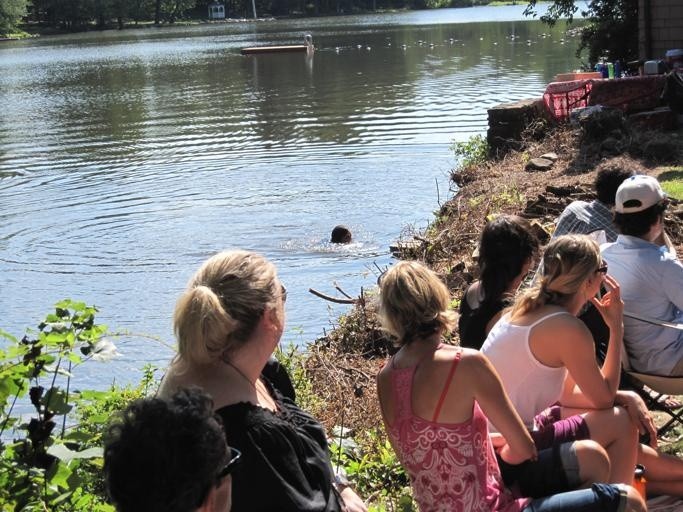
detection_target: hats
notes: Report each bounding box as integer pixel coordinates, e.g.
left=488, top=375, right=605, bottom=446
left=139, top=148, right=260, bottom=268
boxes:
left=614, top=174, right=663, bottom=214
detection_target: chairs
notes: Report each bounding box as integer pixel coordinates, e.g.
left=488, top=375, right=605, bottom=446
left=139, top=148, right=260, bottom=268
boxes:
left=596, top=287, right=683, bottom=445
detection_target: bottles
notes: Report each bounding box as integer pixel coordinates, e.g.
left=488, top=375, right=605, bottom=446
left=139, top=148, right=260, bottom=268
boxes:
left=632, top=464, right=647, bottom=506
left=595, top=61, right=621, bottom=78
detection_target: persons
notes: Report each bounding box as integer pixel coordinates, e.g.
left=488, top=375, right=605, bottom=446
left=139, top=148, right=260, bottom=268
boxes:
left=151, top=247, right=370, bottom=512
left=102, top=385, right=241, bottom=512
left=455, top=213, right=681, bottom=504
left=548, top=156, right=682, bottom=411
left=375, top=261, right=650, bottom=512
left=480, top=233, right=658, bottom=488
left=329, top=224, right=352, bottom=243
left=594, top=173, right=682, bottom=379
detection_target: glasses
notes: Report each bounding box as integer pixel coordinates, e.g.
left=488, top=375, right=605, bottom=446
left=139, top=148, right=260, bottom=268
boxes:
left=215, top=447, right=242, bottom=481
left=595, top=259, right=608, bottom=275
left=274, top=285, right=288, bottom=302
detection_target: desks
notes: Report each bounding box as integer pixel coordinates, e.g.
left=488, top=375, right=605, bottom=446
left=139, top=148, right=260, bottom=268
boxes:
left=543, top=75, right=667, bottom=123
